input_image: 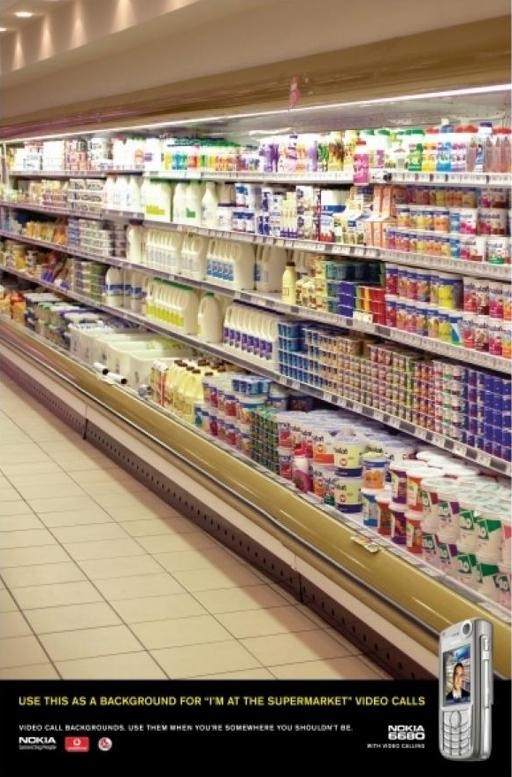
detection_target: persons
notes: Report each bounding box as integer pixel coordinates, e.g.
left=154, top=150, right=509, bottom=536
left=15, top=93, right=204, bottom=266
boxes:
left=445, top=662, right=470, bottom=704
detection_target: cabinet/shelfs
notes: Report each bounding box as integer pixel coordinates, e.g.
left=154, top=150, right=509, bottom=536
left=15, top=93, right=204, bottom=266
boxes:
left=3, top=86, right=509, bottom=678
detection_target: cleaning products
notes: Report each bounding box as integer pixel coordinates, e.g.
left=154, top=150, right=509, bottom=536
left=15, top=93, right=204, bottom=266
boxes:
left=102, top=122, right=512, bottom=618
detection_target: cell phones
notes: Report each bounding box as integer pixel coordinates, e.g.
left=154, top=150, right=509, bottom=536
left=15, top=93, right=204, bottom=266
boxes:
left=439, top=616, right=493, bottom=761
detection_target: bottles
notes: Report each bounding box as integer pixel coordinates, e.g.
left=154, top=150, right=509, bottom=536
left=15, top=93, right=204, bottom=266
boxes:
left=353, top=140, right=370, bottom=186
left=164, top=143, right=258, bottom=172
left=281, top=262, right=298, bottom=303
